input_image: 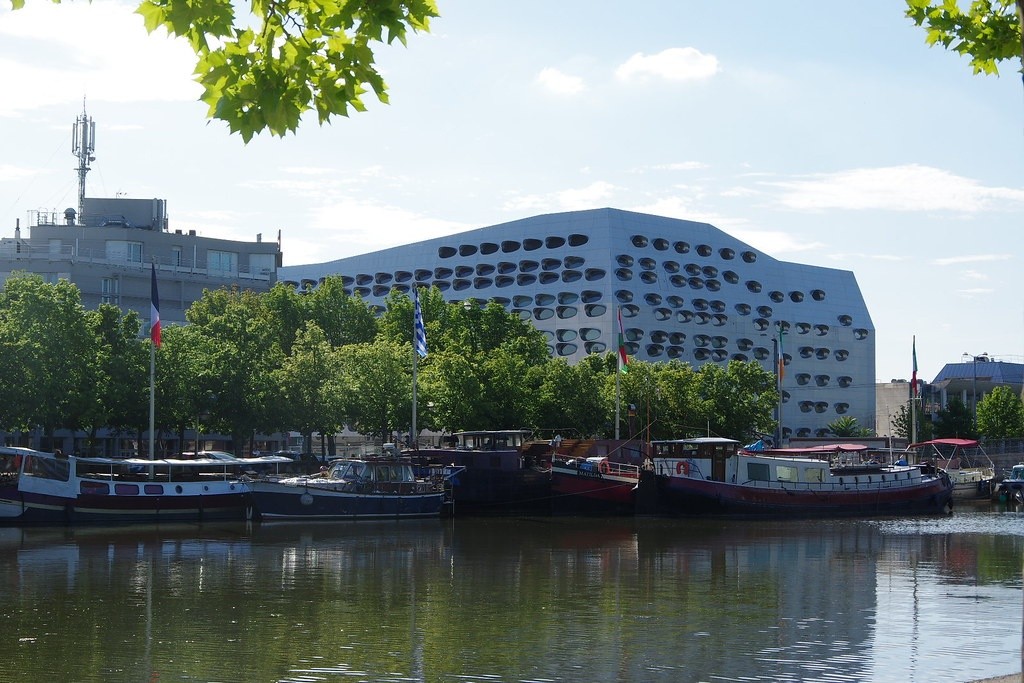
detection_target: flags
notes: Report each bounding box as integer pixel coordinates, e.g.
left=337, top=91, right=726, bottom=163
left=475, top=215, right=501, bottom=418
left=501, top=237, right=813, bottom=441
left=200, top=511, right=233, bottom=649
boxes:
left=414, top=288, right=428, bottom=359
left=618, top=309, right=628, bottom=375
left=151, top=262, right=161, bottom=348
left=912, top=337, right=919, bottom=396
left=778, top=332, right=784, bottom=383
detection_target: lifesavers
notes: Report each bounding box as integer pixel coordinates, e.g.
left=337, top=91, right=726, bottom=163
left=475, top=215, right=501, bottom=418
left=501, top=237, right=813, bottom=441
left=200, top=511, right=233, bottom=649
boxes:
left=599, top=460, right=611, bottom=475
left=676, top=460, right=689, bottom=475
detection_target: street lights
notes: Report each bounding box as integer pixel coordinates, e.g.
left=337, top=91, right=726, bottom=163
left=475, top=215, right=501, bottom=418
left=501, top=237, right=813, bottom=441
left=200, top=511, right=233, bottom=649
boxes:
left=963, top=351, right=988, bottom=434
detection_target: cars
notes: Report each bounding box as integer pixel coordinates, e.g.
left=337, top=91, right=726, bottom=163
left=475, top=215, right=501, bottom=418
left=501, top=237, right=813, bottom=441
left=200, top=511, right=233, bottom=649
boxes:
left=168, top=449, right=241, bottom=461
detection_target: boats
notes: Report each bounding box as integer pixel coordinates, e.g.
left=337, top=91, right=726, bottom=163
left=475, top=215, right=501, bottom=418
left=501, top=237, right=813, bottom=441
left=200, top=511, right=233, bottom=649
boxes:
left=629, top=435, right=957, bottom=513
left=0, top=447, right=300, bottom=517
left=549, top=455, right=639, bottom=502
left=240, top=428, right=641, bottom=517
left=887, top=437, right=999, bottom=500
left=1001, top=464, right=1024, bottom=499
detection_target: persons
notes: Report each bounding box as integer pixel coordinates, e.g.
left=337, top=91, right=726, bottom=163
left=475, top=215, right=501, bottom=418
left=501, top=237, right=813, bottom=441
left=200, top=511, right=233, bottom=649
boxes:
left=320, top=465, right=329, bottom=477
